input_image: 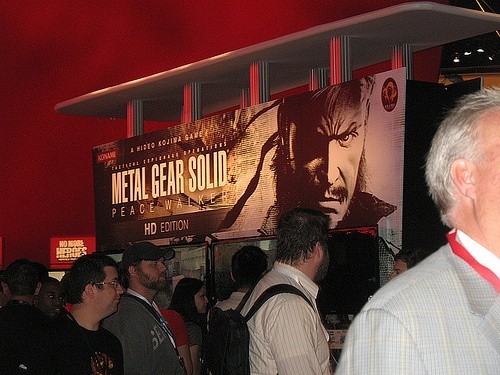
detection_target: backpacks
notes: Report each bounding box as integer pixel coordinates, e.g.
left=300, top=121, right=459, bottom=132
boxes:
left=204, top=270, right=315, bottom=375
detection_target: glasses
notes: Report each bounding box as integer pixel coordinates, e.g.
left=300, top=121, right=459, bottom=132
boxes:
left=94, top=279, right=118, bottom=289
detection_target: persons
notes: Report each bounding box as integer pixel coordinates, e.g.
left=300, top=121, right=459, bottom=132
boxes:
left=257, top=73, right=398, bottom=235
left=0, top=208, right=332, bottom=375
left=335, top=85, right=500, bottom=375
left=388, top=247, right=430, bottom=279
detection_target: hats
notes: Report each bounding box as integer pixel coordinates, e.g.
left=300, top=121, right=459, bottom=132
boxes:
left=121, top=242, right=176, bottom=265
